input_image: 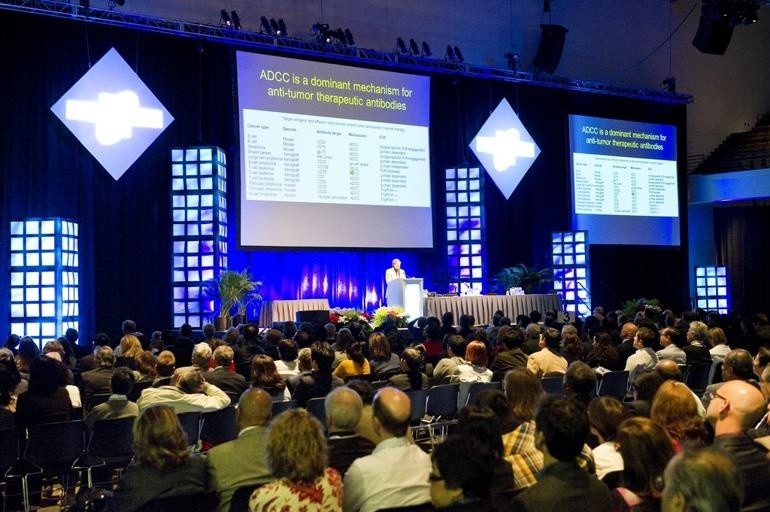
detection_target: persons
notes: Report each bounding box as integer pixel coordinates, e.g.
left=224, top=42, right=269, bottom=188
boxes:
left=2, top=306, right=769, bottom=512
left=385, top=258, right=406, bottom=307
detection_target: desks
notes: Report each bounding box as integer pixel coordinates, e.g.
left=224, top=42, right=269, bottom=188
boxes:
left=260, top=299, right=331, bottom=323
left=424, top=292, right=564, bottom=326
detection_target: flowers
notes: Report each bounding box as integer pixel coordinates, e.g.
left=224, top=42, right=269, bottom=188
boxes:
left=331, top=307, right=369, bottom=326
left=373, top=306, right=411, bottom=328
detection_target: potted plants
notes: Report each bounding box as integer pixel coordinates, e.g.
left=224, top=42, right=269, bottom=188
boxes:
left=209, top=270, right=253, bottom=328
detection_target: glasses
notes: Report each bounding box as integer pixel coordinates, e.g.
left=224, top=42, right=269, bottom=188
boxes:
left=428, top=474, right=445, bottom=482
left=614, top=442, right=625, bottom=453
left=660, top=333, right=667, bottom=336
left=709, top=390, right=725, bottom=402
left=655, top=477, right=666, bottom=491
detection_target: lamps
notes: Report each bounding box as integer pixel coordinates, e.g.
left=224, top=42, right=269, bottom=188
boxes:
left=444, top=44, right=455, bottom=60
left=259, top=15, right=272, bottom=36
left=218, top=9, right=231, bottom=26
left=396, top=36, right=408, bottom=54
left=269, top=17, right=281, bottom=36
left=408, top=38, right=420, bottom=55
left=315, top=17, right=358, bottom=47
left=230, top=10, right=241, bottom=30
left=279, top=18, right=287, bottom=36
left=421, top=40, right=431, bottom=58
left=454, top=45, right=464, bottom=62
left=108, top=0, right=125, bottom=10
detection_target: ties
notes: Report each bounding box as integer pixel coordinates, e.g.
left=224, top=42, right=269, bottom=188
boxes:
left=396, top=270, right=399, bottom=279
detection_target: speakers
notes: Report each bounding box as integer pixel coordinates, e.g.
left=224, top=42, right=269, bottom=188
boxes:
left=272, top=322, right=298, bottom=333
left=296, top=310, right=329, bottom=330
left=691, top=0, right=734, bottom=55
left=532, top=23, right=568, bottom=75
